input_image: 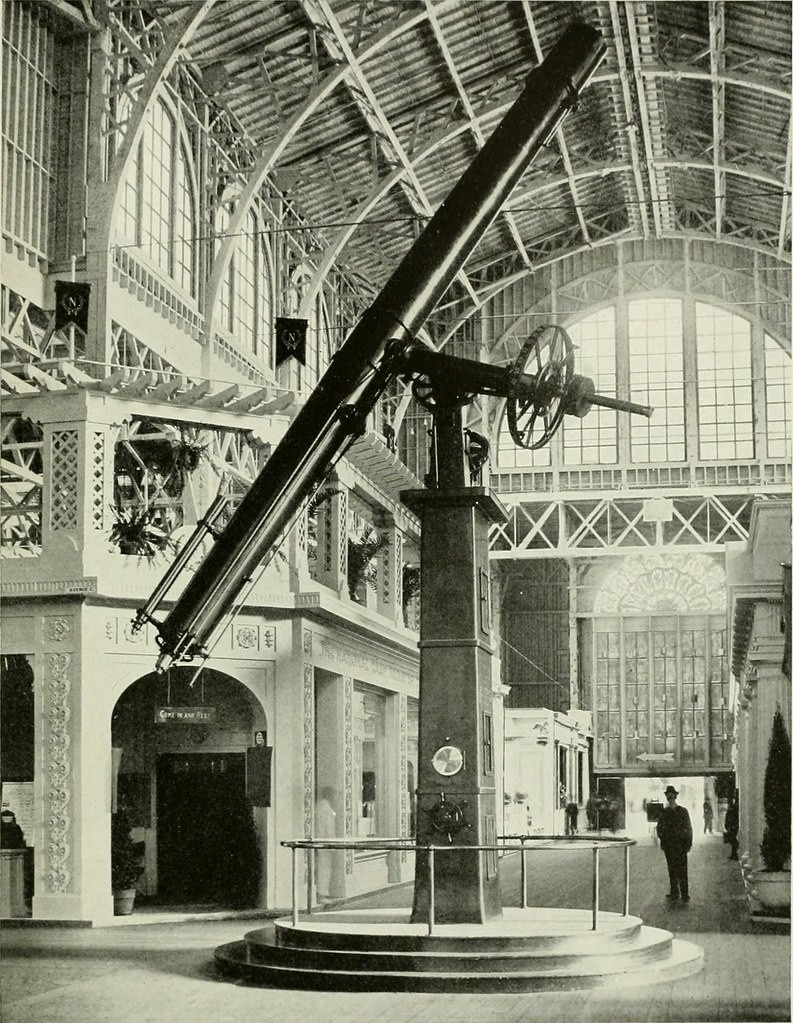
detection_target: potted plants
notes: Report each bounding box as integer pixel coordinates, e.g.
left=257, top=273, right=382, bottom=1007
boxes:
left=753, top=699, right=791, bottom=912
left=505, top=791, right=512, bottom=805
left=515, top=789, right=529, bottom=804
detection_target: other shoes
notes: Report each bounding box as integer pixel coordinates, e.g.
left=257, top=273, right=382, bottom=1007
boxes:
left=728, top=856, right=737, bottom=860
left=666, top=893, right=690, bottom=901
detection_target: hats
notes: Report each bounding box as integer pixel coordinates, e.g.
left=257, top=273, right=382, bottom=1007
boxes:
left=664, top=786, right=679, bottom=794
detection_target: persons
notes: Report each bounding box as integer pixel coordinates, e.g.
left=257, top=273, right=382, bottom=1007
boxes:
left=703, top=797, right=740, bottom=861
left=656, top=786, right=692, bottom=903
